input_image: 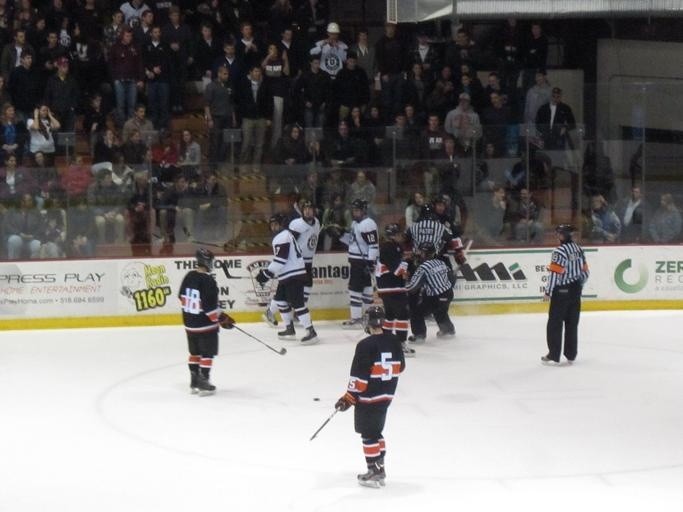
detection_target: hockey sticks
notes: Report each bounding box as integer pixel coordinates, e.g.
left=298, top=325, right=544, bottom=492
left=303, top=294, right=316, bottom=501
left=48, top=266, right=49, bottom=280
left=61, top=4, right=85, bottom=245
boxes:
left=222, top=263, right=277, bottom=280
left=232, top=325, right=286, bottom=355
left=310, top=405, right=341, bottom=440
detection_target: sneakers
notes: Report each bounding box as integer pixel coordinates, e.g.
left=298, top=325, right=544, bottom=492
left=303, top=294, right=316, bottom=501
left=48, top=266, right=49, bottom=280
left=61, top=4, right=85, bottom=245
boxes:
left=402, top=344, right=415, bottom=356
left=342, top=317, right=363, bottom=326
left=437, top=329, right=456, bottom=337
left=542, top=354, right=559, bottom=362
left=408, top=334, right=427, bottom=341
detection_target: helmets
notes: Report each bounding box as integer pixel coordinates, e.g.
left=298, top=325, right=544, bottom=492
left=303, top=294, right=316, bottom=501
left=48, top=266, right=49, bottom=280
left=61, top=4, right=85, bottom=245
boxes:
left=421, top=195, right=451, bottom=219
left=352, top=199, right=368, bottom=219
left=269, top=213, right=284, bottom=234
left=418, top=241, right=436, bottom=261
left=386, top=223, right=400, bottom=240
left=363, top=306, right=385, bottom=334
left=301, top=200, right=315, bottom=218
left=327, top=22, right=340, bottom=33
left=196, top=247, right=213, bottom=266
left=556, top=224, right=573, bottom=242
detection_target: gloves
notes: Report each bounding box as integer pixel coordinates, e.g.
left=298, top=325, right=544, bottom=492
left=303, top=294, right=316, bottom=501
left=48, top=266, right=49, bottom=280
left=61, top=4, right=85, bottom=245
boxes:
left=255, top=271, right=268, bottom=284
left=453, top=250, right=466, bottom=265
left=363, top=263, right=376, bottom=276
left=218, top=313, right=234, bottom=329
left=333, top=397, right=349, bottom=411
left=327, top=224, right=342, bottom=238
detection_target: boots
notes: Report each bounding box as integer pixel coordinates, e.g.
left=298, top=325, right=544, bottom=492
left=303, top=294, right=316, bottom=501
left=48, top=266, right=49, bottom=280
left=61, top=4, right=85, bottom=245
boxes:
left=262, top=311, right=278, bottom=327
left=358, top=460, right=386, bottom=480
left=300, top=325, right=317, bottom=341
left=190, top=371, right=215, bottom=391
left=278, top=320, right=296, bottom=336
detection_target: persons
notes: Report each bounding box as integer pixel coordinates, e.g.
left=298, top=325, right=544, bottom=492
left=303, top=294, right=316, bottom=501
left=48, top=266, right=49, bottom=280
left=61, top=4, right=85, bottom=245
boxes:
left=376, top=220, right=418, bottom=356
left=341, top=199, right=381, bottom=328
left=177, top=247, right=237, bottom=395
left=260, top=198, right=324, bottom=326
left=253, top=215, right=320, bottom=345
left=334, top=306, right=406, bottom=485
left=539, top=223, right=591, bottom=364
left=1, top=0, right=682, bottom=262
left=405, top=241, right=457, bottom=341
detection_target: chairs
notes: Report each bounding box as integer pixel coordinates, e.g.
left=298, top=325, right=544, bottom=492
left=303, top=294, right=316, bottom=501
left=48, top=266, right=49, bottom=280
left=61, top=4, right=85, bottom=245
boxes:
left=2, top=81, right=604, bottom=257
